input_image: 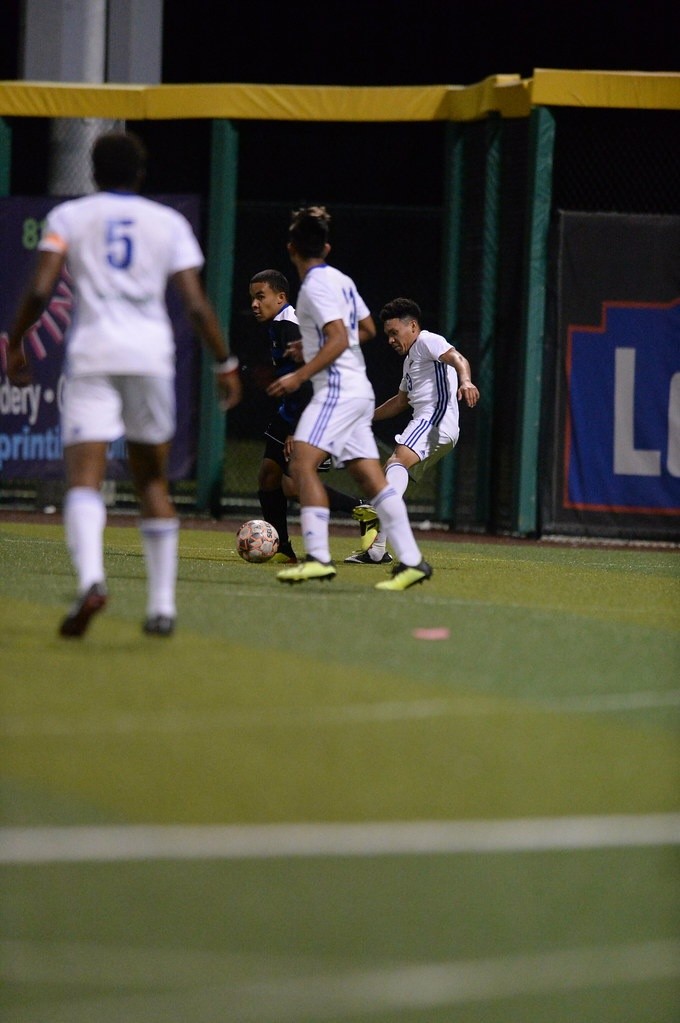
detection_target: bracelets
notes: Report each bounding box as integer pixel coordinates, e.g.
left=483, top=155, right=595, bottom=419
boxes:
left=212, top=355, right=239, bottom=376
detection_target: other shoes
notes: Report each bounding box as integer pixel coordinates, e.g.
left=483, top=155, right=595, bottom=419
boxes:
left=60, top=584, right=105, bottom=635
left=144, top=616, right=175, bottom=635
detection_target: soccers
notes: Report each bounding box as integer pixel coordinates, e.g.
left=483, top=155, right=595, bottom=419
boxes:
left=235, top=519, right=279, bottom=564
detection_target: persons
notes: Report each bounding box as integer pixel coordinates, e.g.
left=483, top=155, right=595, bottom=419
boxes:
left=343, top=297, right=480, bottom=564
left=7, top=135, right=243, bottom=638
left=250, top=269, right=380, bottom=564
left=265, top=205, right=433, bottom=591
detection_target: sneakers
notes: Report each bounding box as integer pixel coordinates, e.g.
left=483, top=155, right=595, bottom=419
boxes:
left=352, top=497, right=379, bottom=551
left=352, top=504, right=378, bottom=522
left=268, top=542, right=296, bottom=564
left=276, top=553, right=337, bottom=582
left=344, top=551, right=392, bottom=563
left=374, top=557, right=432, bottom=592
